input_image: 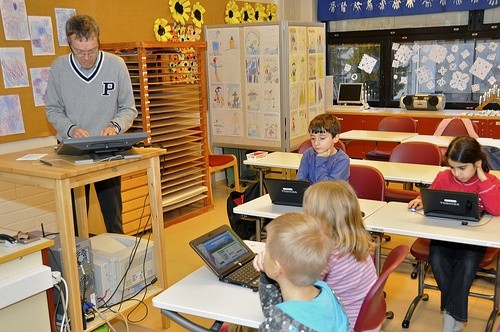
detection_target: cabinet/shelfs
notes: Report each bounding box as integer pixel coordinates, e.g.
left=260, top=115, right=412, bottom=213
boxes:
left=100, top=40, right=216, bottom=228
left=0, top=226, right=58, bottom=332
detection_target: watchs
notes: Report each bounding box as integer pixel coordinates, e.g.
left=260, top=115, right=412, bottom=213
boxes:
left=108, top=125, right=119, bottom=134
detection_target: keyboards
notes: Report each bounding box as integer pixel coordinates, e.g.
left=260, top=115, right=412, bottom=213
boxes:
left=332, top=105, right=363, bottom=111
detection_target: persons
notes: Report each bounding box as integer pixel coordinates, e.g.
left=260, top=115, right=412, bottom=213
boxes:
left=253, top=212, right=348, bottom=332
left=295, top=114, right=350, bottom=185
left=45, top=15, right=138, bottom=237
left=408, top=136, right=500, bottom=332
left=302, top=181, right=379, bottom=332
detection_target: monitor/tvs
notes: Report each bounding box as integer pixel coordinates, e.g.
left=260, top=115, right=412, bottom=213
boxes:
left=336, top=83, right=364, bottom=104
left=55, top=133, right=147, bottom=158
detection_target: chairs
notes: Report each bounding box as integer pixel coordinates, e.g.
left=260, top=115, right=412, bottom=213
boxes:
left=384, top=141, right=443, bottom=202
left=379, top=117, right=417, bottom=133
left=402, top=236, right=500, bottom=332
left=436, top=117, right=480, bottom=138
left=208, top=146, right=239, bottom=201
left=354, top=244, right=409, bottom=332
left=346, top=164, right=386, bottom=201
left=299, top=138, right=346, bottom=155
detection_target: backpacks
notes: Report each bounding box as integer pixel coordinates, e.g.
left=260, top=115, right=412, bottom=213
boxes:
left=227, top=180, right=269, bottom=240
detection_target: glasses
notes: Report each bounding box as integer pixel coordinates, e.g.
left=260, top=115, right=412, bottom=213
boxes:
left=69, top=40, right=100, bottom=56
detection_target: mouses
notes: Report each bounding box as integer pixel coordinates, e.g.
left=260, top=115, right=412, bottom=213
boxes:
left=409, top=205, right=419, bottom=212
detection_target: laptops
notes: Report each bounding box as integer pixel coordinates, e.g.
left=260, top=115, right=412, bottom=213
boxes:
left=189, top=224, right=262, bottom=290
left=420, top=188, right=485, bottom=222
left=262, top=179, right=312, bottom=206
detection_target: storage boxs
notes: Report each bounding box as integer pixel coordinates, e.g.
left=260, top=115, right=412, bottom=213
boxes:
left=246, top=150, right=268, bottom=160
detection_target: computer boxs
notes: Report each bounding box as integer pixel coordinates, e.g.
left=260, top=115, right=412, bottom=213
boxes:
left=28, top=230, right=97, bottom=326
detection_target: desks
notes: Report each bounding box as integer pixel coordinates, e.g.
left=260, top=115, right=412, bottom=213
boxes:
left=0, top=141, right=170, bottom=332
left=152, top=106, right=500, bottom=332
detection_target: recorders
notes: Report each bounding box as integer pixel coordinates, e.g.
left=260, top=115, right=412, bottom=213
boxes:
left=399, top=93, right=446, bottom=110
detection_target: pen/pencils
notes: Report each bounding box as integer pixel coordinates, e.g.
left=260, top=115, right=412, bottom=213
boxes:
left=40, top=160, right=52, bottom=166
